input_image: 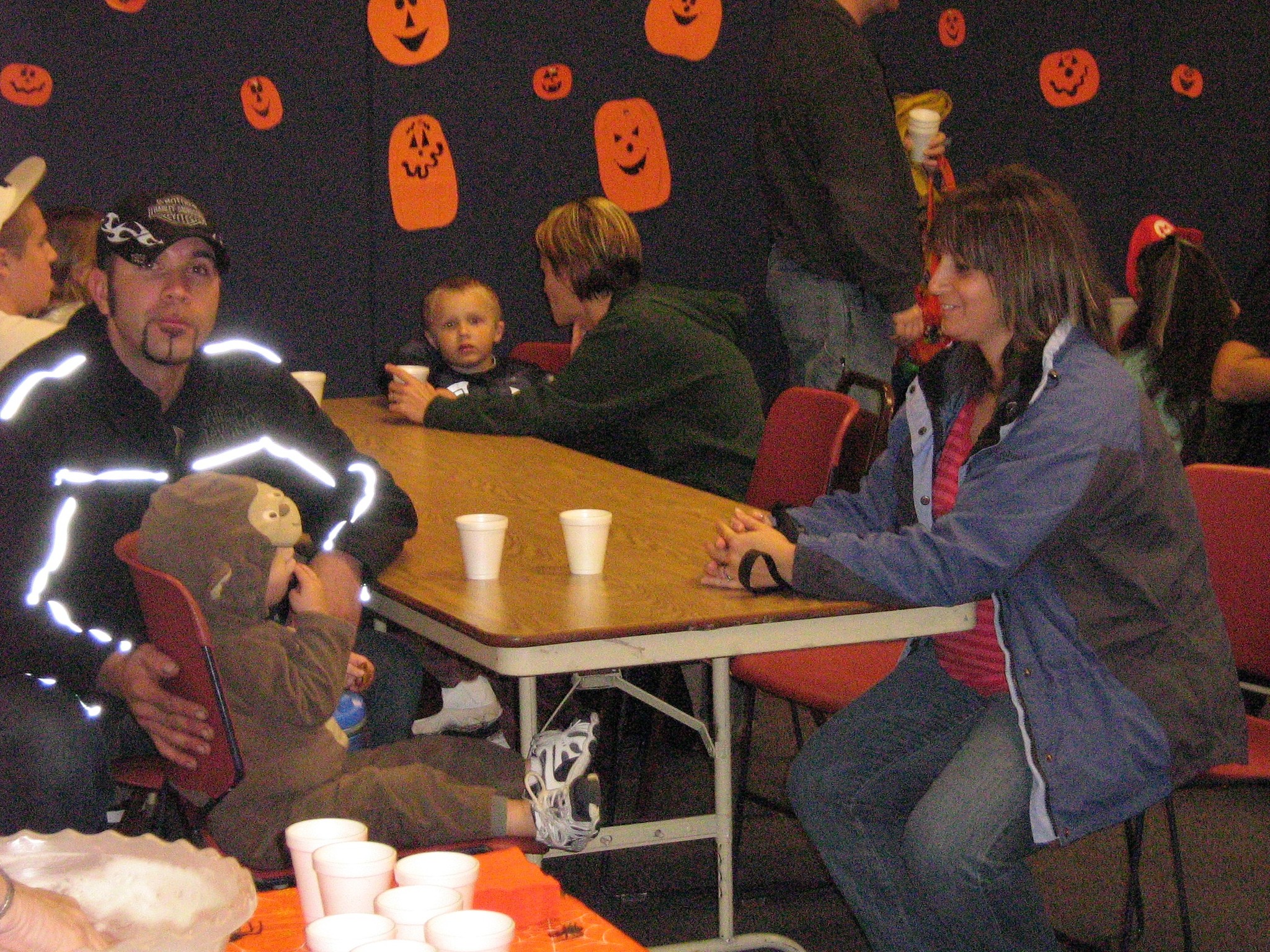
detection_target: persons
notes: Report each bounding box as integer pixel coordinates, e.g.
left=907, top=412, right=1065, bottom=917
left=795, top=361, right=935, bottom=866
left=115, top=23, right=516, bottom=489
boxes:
left=696, top=169, right=1246, bottom=952
left=0, top=155, right=103, bottom=373
left=740, top=0, right=947, bottom=419
left=0, top=193, right=425, bottom=834
left=425, top=274, right=550, bottom=397
left=140, top=472, right=607, bottom=870
left=383, top=197, right=767, bottom=736
left=1118, top=212, right=1270, bottom=467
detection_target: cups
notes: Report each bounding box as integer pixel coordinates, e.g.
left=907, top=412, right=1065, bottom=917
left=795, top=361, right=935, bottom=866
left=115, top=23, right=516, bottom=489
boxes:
left=284, top=817, right=368, bottom=925
left=313, top=841, right=397, bottom=914
left=393, top=365, right=430, bottom=384
left=291, top=372, right=326, bottom=408
left=559, top=508, right=612, bottom=574
left=456, top=515, right=508, bottom=579
left=394, top=851, right=480, bottom=909
left=425, top=909, right=517, bottom=952
left=306, top=914, right=395, bottom=952
left=348, top=941, right=435, bottom=951
left=374, top=886, right=463, bottom=940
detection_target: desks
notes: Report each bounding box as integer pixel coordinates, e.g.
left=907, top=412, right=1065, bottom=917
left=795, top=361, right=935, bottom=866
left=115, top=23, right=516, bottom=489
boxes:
left=322, top=397, right=978, bottom=952
left=221, top=845, right=650, bottom=952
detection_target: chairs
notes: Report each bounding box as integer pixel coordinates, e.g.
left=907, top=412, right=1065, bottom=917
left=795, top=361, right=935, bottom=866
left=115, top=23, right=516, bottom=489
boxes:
left=115, top=527, right=550, bottom=887
left=592, top=368, right=1270, bottom=952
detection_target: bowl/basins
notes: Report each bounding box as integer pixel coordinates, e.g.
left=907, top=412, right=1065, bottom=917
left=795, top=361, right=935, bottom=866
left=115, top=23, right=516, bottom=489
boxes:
left=0, top=828, right=258, bottom=951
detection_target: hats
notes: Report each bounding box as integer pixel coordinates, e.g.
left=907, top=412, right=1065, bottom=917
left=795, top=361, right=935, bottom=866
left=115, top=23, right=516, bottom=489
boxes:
left=1126, top=214, right=1203, bottom=306
left=98, top=191, right=230, bottom=273
left=0, top=155, right=47, bottom=232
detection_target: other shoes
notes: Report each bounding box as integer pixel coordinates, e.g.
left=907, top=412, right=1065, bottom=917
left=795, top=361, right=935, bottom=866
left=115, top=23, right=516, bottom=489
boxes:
left=521, top=773, right=601, bottom=853
left=411, top=699, right=502, bottom=734
left=486, top=729, right=512, bottom=750
left=530, top=712, right=601, bottom=796
